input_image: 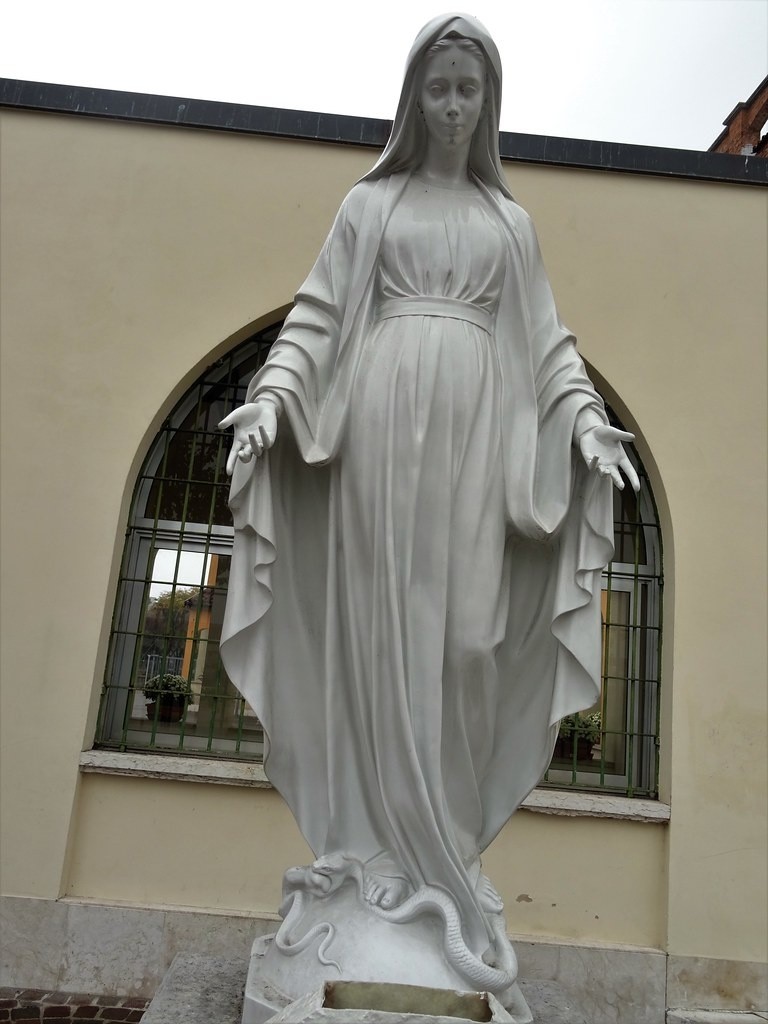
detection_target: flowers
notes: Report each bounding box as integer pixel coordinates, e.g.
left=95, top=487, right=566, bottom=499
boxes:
left=143, top=674, right=194, bottom=706
left=559, top=713, right=605, bottom=743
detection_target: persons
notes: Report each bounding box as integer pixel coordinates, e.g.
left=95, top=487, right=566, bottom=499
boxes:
left=217, top=11, right=642, bottom=906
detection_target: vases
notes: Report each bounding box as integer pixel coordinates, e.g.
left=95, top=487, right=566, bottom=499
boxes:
left=147, top=703, right=184, bottom=722
left=555, top=738, right=595, bottom=760
